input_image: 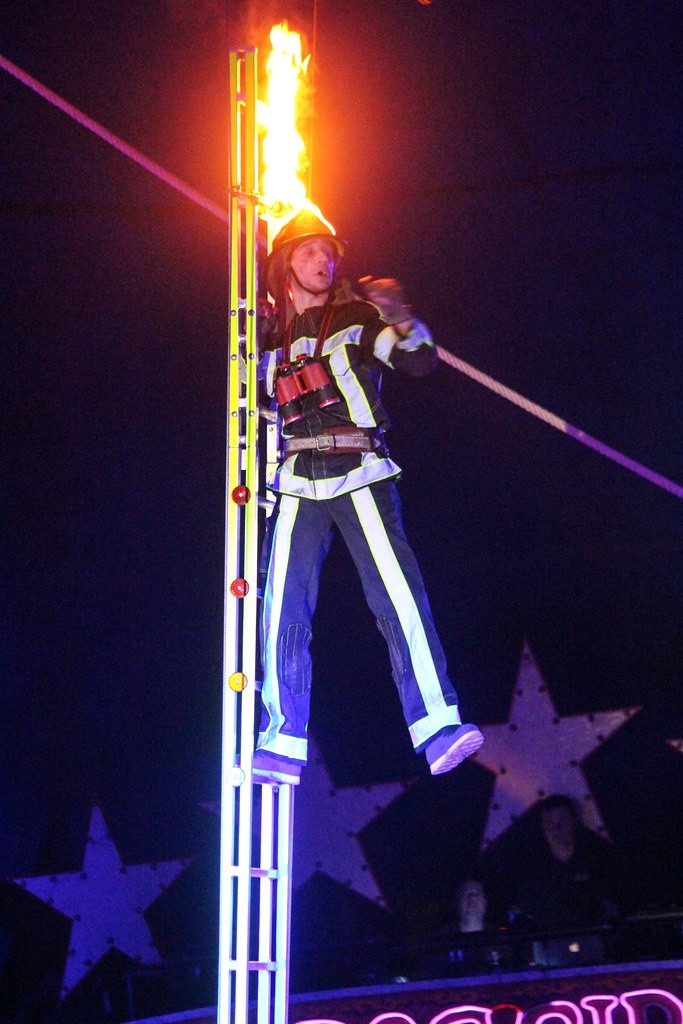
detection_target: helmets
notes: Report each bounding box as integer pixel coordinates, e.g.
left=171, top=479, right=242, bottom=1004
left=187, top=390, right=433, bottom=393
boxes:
left=262, top=211, right=346, bottom=299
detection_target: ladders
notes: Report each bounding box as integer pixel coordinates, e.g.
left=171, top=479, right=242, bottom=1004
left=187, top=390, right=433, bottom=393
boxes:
left=216, top=38, right=292, bottom=1024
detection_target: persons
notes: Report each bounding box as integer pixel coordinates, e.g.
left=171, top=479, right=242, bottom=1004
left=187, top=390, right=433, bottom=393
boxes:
left=233, top=209, right=485, bottom=785
left=425, top=793, right=623, bottom=976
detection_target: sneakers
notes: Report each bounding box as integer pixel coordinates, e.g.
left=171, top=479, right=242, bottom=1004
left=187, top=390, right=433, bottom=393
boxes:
left=237, top=750, right=304, bottom=785
left=426, top=723, right=484, bottom=776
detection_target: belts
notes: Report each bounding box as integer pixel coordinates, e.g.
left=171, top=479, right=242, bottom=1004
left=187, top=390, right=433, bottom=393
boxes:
left=282, top=434, right=381, bottom=453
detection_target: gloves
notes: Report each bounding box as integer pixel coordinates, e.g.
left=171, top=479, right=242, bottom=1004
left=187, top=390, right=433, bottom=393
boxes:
left=363, top=279, right=411, bottom=325
